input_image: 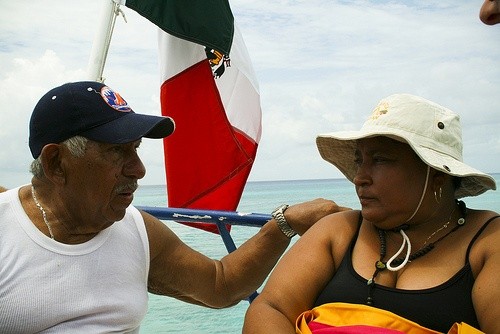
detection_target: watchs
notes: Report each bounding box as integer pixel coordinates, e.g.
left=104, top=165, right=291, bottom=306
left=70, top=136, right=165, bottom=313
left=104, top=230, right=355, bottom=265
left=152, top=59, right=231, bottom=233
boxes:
left=271, top=204, right=297, bottom=238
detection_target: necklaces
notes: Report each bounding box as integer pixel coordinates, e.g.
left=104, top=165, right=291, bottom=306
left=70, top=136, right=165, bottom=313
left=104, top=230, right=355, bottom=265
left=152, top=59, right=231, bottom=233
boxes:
left=31, top=185, right=54, bottom=239
left=366, top=200, right=467, bottom=306
left=420, top=205, right=457, bottom=250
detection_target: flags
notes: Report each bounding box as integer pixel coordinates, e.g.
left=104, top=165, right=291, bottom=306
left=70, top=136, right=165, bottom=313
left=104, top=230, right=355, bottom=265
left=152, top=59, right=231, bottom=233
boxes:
left=115, top=0, right=262, bottom=236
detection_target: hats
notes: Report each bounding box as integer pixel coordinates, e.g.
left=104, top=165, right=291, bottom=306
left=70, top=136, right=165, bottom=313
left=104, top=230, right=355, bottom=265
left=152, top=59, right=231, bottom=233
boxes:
left=316, top=93, right=497, bottom=199
left=29, top=81, right=176, bottom=160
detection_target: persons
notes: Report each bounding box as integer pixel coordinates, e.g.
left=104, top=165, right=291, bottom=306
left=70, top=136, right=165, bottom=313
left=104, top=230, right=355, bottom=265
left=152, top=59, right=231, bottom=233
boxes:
left=0, top=82, right=355, bottom=334
left=244, top=94, right=500, bottom=334
